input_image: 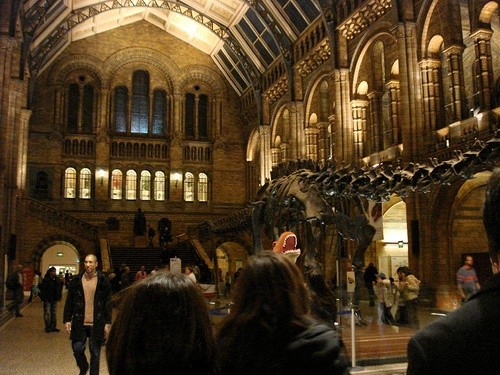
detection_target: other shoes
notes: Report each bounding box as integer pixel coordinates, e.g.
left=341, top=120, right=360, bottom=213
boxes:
left=45, top=328, right=50, bottom=333
left=15, top=313, right=23, bottom=317
left=50, top=328, right=60, bottom=332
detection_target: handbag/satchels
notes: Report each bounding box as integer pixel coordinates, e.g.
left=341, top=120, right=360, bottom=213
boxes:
left=33, top=286, right=40, bottom=295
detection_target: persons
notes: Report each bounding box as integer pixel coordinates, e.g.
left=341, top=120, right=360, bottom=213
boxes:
left=63, top=255, right=123, bottom=375
left=353, top=265, right=365, bottom=314
left=214, top=250, right=351, bottom=375
left=136, top=266, right=146, bottom=281
left=151, top=266, right=159, bottom=275
left=305, top=262, right=338, bottom=325
left=148, top=225, right=156, bottom=242
left=372, top=266, right=421, bottom=329
left=136, top=208, right=144, bottom=236
left=364, top=263, right=379, bottom=309
left=28, top=267, right=65, bottom=333
left=5, top=264, right=24, bottom=317
left=457, top=257, right=481, bottom=304
left=407, top=168, right=500, bottom=375
left=119, top=264, right=130, bottom=289
left=106, top=272, right=217, bottom=375
left=184, top=261, right=201, bottom=285
left=224, top=271, right=234, bottom=299
left=233, top=268, right=243, bottom=285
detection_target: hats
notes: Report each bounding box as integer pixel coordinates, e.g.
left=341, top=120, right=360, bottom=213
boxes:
left=375, top=273, right=386, bottom=279
